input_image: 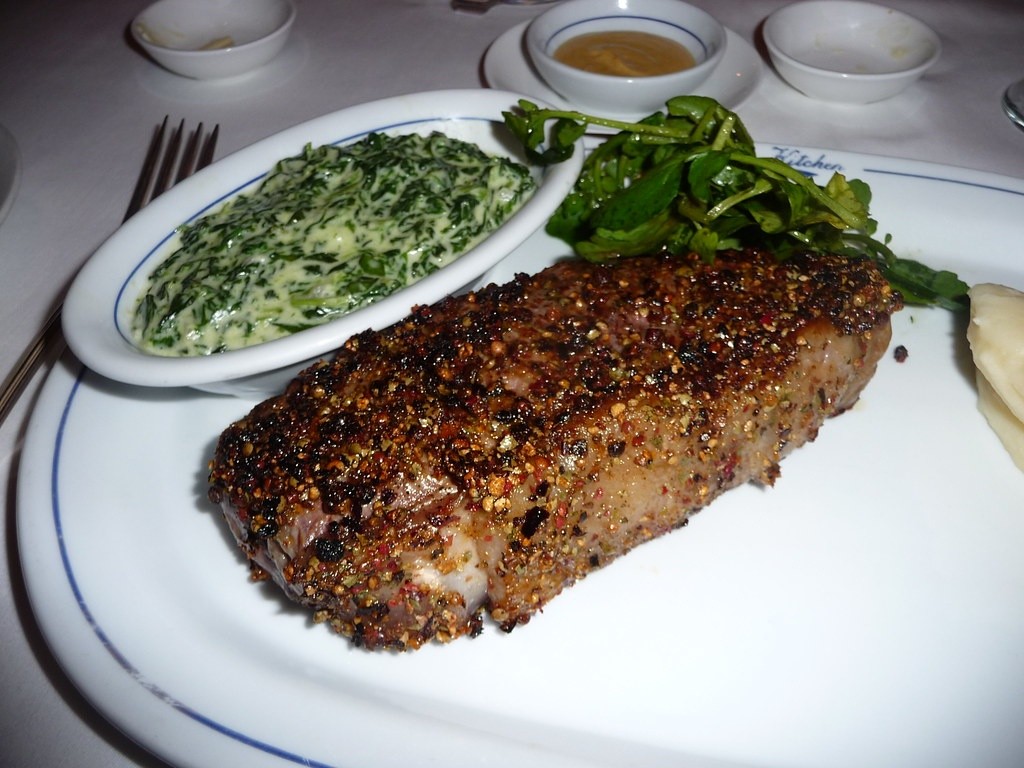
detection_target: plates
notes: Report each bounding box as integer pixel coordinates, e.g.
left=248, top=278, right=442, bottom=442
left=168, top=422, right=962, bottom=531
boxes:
left=11, top=139, right=1024, bottom=766
left=481, top=17, right=764, bottom=135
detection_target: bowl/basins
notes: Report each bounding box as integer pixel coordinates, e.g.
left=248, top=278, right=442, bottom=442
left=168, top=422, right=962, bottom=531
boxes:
left=129, top=0, right=298, bottom=81
left=760, top=0, right=942, bottom=107
left=60, top=87, right=586, bottom=398
left=525, top=0, right=729, bottom=117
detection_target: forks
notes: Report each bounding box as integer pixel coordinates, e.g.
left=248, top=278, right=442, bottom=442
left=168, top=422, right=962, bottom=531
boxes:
left=1, top=112, right=221, bottom=427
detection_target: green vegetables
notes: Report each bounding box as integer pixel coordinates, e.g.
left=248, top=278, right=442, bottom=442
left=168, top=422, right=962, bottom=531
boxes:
left=508, top=92, right=972, bottom=318
left=128, top=130, right=538, bottom=357
